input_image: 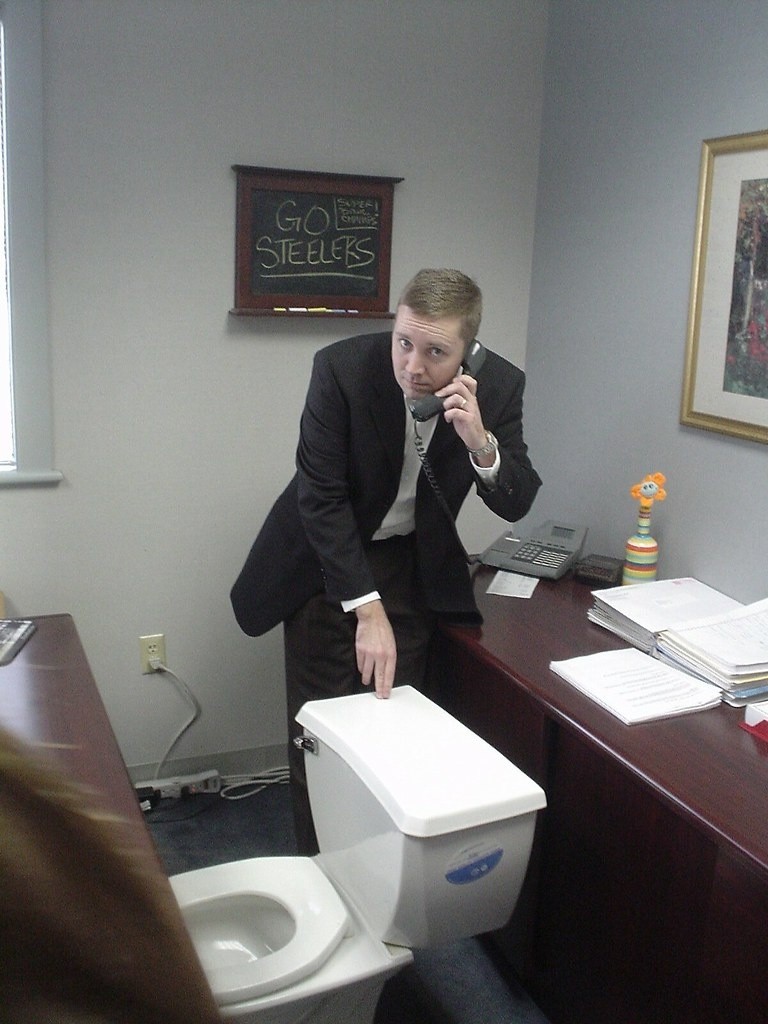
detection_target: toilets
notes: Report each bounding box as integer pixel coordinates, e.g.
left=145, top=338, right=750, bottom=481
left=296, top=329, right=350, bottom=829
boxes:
left=169, top=684, right=548, bottom=1024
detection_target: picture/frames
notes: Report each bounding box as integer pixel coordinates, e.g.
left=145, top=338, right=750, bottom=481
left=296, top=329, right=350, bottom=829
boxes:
left=679, top=131, right=768, bottom=446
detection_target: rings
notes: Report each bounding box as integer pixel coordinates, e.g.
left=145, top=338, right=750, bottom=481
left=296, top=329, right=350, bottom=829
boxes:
left=459, top=398, right=467, bottom=409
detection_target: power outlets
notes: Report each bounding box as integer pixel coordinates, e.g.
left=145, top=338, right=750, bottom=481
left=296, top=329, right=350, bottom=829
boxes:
left=140, top=634, right=166, bottom=674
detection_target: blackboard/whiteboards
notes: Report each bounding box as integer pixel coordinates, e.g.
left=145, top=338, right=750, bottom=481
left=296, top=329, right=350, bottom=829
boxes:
left=227, top=162, right=406, bottom=320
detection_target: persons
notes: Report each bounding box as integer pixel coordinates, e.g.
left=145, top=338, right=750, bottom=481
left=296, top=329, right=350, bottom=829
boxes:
left=283, top=267, right=543, bottom=858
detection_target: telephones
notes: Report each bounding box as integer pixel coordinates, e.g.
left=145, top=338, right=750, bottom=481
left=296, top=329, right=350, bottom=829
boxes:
left=404, top=335, right=486, bottom=423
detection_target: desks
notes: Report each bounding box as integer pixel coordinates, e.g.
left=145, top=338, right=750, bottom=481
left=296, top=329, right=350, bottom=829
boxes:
left=0, top=613, right=224, bottom=1024
left=420, top=557, right=768, bottom=1024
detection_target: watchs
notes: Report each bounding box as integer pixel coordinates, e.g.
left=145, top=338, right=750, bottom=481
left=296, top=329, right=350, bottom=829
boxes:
left=466, top=430, right=499, bottom=458
